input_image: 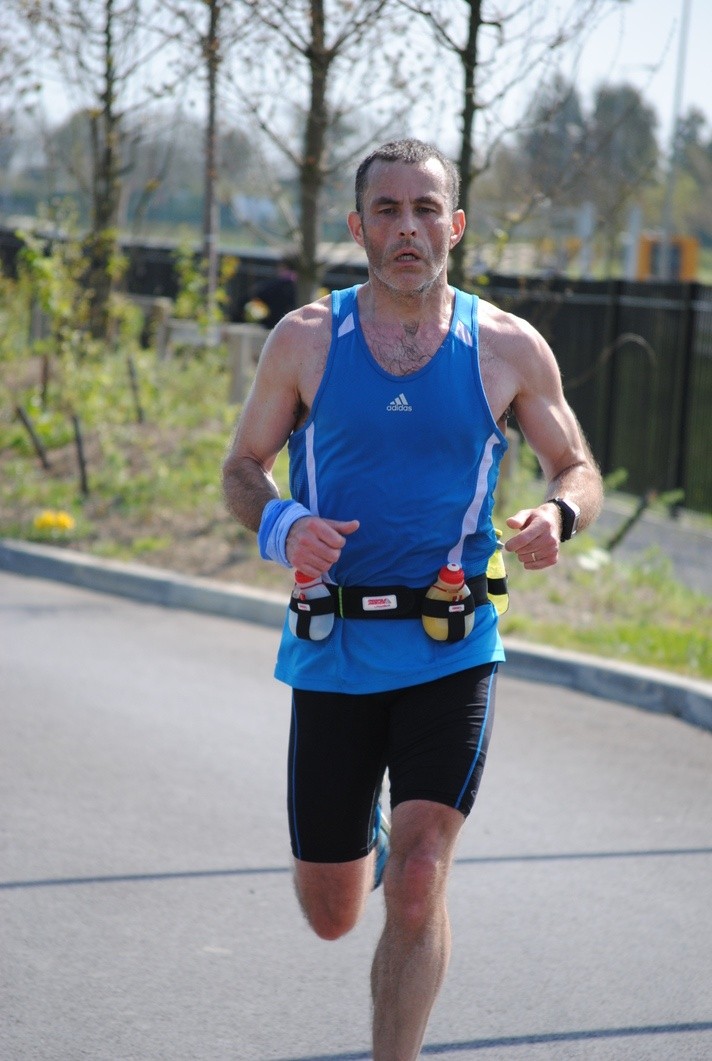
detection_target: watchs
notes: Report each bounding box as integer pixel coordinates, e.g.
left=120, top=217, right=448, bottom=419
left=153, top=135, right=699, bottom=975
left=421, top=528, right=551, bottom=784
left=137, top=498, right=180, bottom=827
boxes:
left=545, top=497, right=581, bottom=543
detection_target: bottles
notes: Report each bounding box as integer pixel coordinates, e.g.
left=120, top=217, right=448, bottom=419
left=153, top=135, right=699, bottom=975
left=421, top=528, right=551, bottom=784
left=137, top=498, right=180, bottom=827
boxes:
left=486, top=528, right=510, bottom=616
left=422, top=563, right=475, bottom=641
left=288, top=570, right=334, bottom=641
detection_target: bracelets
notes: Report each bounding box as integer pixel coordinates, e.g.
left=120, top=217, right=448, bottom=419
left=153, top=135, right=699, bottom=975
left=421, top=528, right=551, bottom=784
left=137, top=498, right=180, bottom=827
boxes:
left=257, top=497, right=313, bottom=569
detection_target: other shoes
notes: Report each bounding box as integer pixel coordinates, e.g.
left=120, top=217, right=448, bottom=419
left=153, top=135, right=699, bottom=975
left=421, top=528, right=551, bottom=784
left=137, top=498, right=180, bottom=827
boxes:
left=372, top=795, right=391, bottom=890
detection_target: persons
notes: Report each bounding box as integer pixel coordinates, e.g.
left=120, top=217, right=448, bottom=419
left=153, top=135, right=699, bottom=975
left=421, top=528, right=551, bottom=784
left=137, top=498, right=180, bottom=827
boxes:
left=221, top=138, right=605, bottom=1061
